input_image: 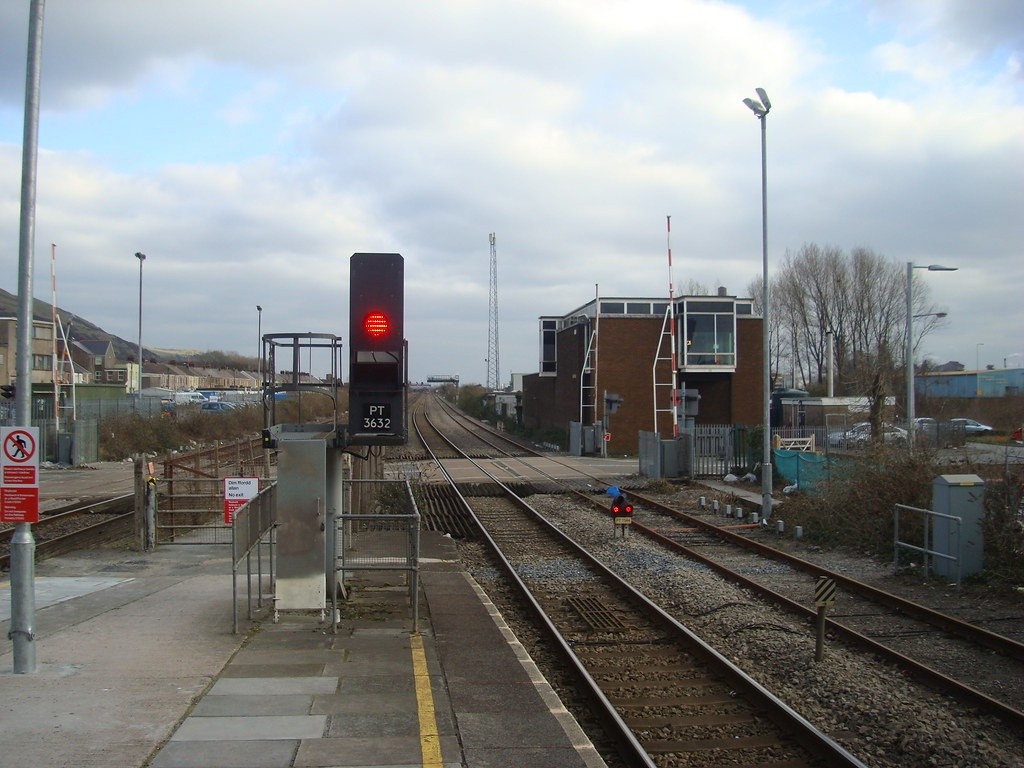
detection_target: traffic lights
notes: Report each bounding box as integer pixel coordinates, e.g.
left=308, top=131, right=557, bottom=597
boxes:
left=613, top=496, right=632, bottom=517
left=349, top=253, right=404, bottom=438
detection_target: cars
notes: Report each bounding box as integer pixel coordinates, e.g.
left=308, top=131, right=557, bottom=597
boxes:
left=944, top=418, right=993, bottom=434
left=829, top=422, right=908, bottom=446
left=200, top=401, right=238, bottom=414
left=915, top=417, right=938, bottom=442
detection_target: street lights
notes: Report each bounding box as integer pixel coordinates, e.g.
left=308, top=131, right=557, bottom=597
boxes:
left=257, top=305, right=262, bottom=403
left=742, top=88, right=773, bottom=524
left=907, top=261, right=958, bottom=453
left=308, top=332, right=312, bottom=383
left=976, top=343, right=985, bottom=404
left=134, top=252, right=146, bottom=400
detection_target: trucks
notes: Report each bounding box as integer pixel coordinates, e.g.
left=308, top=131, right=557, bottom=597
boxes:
left=174, top=392, right=208, bottom=404
